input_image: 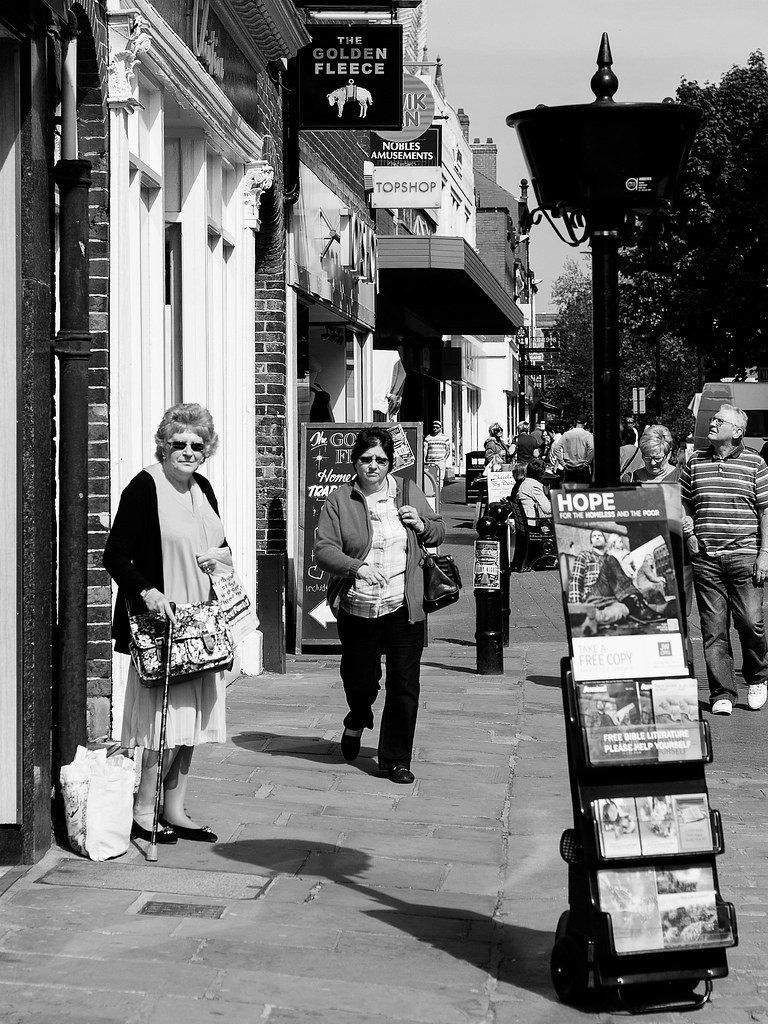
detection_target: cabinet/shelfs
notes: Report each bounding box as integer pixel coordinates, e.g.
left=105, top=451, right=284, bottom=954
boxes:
left=550, top=480, right=739, bottom=1012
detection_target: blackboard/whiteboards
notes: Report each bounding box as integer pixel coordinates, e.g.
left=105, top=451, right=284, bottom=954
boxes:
left=296, top=420, right=424, bottom=646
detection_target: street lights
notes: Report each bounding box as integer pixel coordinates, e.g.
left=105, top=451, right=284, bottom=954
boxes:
left=506, top=31, right=703, bottom=487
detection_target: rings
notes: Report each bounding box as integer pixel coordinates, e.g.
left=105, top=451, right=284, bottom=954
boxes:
left=203, top=562, right=208, bottom=568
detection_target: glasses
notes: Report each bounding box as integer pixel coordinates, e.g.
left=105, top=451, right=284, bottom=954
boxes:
left=433, top=426, right=439, bottom=429
left=642, top=453, right=666, bottom=463
left=497, top=429, right=503, bottom=432
left=709, top=417, right=736, bottom=427
left=359, top=456, right=388, bottom=465
left=167, top=441, right=205, bottom=451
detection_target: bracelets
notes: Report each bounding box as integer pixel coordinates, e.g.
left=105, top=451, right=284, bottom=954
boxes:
left=686, top=533, right=694, bottom=542
left=759, top=547, right=768, bottom=553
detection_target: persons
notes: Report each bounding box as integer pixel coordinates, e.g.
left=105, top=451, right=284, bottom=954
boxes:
left=508, top=420, right=539, bottom=464
left=530, top=422, right=555, bottom=463
left=516, top=458, right=554, bottom=532
left=568, top=529, right=676, bottom=625
left=511, top=461, right=531, bottom=503
left=626, top=418, right=639, bottom=448
left=553, top=414, right=595, bottom=483
left=483, top=422, right=511, bottom=472
left=618, top=427, right=645, bottom=484
left=619, top=424, right=694, bottom=666
left=596, top=695, right=668, bottom=838
left=313, top=426, right=447, bottom=786
left=679, top=403, right=768, bottom=715
left=424, top=421, right=451, bottom=504
left=546, top=423, right=566, bottom=489
left=102, top=402, right=234, bottom=845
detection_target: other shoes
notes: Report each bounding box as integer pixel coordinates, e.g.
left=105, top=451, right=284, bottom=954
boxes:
left=159, top=813, right=218, bottom=842
left=388, top=766, right=415, bottom=783
left=341, top=728, right=364, bottom=761
left=132, top=818, right=177, bottom=844
left=629, top=599, right=667, bottom=624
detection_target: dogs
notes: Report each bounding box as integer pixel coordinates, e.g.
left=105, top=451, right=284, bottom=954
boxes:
left=326, top=84, right=374, bottom=119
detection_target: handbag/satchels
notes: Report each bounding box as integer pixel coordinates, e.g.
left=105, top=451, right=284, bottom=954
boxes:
left=60, top=745, right=136, bottom=862
left=127, top=600, right=234, bottom=688
left=401, top=475, right=462, bottom=613
left=203, top=547, right=261, bottom=648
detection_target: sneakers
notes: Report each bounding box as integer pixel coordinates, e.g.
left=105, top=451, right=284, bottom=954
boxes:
left=747, top=681, right=767, bottom=709
left=712, top=700, right=733, bottom=715
